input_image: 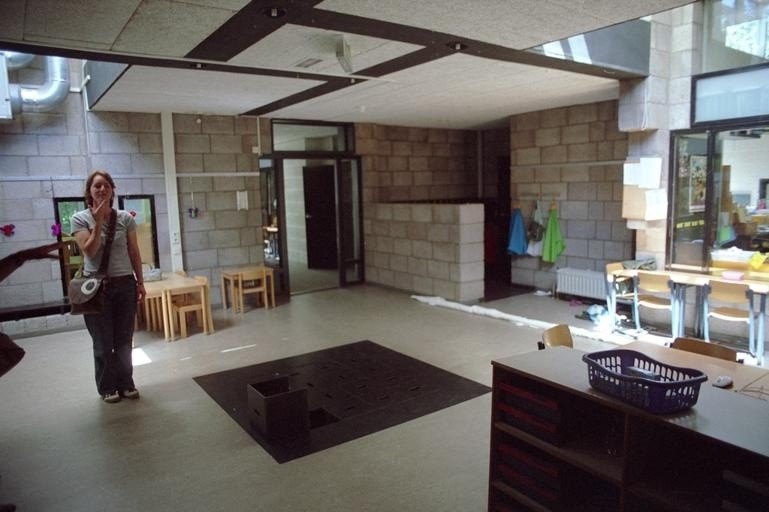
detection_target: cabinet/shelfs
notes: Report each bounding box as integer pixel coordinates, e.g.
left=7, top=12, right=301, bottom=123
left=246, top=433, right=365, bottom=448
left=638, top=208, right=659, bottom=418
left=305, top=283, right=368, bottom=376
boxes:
left=488, top=345, right=769, bottom=511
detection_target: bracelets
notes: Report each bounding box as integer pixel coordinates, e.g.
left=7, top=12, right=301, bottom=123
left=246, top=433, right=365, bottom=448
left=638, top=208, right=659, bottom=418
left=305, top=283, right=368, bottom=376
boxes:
left=138, top=282, right=143, bottom=286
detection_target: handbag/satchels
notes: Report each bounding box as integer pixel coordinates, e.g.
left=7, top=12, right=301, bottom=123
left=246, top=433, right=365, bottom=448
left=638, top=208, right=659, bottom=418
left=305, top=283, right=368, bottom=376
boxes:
left=67, top=275, right=108, bottom=315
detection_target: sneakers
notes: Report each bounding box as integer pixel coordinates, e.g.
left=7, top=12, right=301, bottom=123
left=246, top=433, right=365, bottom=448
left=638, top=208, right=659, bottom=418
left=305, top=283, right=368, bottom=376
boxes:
left=103, top=391, right=122, bottom=403
left=123, top=388, right=140, bottom=400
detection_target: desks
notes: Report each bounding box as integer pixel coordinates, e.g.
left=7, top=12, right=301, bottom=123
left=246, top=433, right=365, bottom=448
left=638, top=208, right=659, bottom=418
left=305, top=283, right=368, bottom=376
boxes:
left=613, top=270, right=768, bottom=365
left=220, top=265, right=276, bottom=310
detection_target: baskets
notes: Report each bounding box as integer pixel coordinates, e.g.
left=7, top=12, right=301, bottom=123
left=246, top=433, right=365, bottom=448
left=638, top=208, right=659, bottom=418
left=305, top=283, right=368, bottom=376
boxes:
left=582, top=348, right=709, bottom=414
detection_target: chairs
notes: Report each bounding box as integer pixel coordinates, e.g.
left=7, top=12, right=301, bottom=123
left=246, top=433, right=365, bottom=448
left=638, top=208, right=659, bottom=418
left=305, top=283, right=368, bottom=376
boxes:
left=605, top=263, right=634, bottom=323
left=703, top=280, right=757, bottom=358
left=635, top=272, right=680, bottom=338
left=234, top=269, right=269, bottom=313
left=135, top=269, right=214, bottom=342
left=542, top=324, right=573, bottom=351
left=671, top=337, right=736, bottom=362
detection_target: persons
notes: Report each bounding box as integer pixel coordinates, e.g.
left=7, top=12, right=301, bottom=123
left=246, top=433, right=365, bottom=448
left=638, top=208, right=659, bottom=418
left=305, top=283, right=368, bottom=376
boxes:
left=70, top=168, right=147, bottom=405
left=0, top=240, right=72, bottom=376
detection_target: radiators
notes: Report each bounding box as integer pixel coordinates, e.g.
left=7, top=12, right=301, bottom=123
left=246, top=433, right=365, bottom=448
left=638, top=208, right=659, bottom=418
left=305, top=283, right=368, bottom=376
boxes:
left=553, top=266, right=633, bottom=305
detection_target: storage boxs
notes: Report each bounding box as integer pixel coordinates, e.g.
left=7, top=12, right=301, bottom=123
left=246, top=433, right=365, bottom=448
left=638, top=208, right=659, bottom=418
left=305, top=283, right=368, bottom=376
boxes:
left=247, top=378, right=310, bottom=446
